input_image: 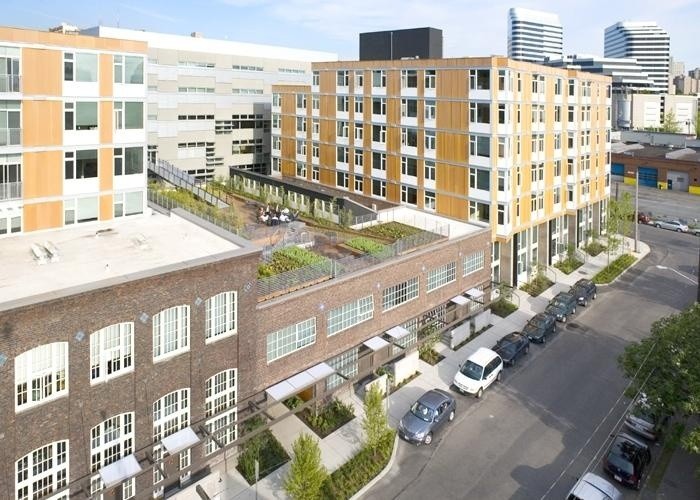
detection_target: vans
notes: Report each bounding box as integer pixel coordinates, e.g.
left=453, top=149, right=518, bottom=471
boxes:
left=454, top=347, right=504, bottom=398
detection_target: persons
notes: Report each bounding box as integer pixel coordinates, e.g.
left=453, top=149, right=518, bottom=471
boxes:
left=258, top=203, right=293, bottom=224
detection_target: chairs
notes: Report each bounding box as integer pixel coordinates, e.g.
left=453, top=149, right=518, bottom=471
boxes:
left=255, top=209, right=299, bottom=226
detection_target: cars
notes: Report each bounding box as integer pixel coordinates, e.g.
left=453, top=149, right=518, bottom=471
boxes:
left=565, top=472, right=622, bottom=500
left=633, top=211, right=651, bottom=224
left=398, top=388, right=456, bottom=446
left=688, top=218, right=700, bottom=237
left=604, top=431, right=652, bottom=491
left=522, top=313, right=557, bottom=344
left=624, top=398, right=673, bottom=440
left=654, top=220, right=689, bottom=233
left=492, top=332, right=530, bottom=366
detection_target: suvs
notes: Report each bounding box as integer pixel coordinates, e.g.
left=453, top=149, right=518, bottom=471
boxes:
left=545, top=292, right=578, bottom=323
left=568, top=279, right=597, bottom=306
